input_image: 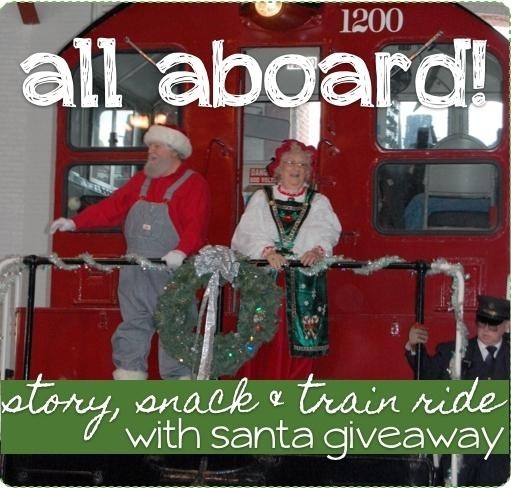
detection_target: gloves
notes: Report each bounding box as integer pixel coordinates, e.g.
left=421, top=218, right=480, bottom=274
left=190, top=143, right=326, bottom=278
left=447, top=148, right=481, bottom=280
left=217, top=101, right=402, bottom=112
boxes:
left=160, top=249, right=187, bottom=273
left=48, top=216, right=77, bottom=236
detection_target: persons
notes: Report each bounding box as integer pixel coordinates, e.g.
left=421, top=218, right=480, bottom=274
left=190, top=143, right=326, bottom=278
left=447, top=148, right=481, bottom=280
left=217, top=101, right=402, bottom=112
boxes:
left=404, top=295, right=510, bottom=486
left=47, top=123, right=210, bottom=382
left=230, top=138, right=341, bottom=271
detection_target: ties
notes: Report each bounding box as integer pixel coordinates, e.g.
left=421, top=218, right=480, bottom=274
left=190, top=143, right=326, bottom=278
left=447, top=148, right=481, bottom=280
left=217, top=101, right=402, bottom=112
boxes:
left=484, top=345, right=497, bottom=375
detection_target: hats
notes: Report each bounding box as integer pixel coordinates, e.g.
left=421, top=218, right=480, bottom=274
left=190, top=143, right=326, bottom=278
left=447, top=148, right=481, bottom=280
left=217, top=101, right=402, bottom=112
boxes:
left=142, top=122, right=193, bottom=160
left=475, top=294, right=510, bottom=326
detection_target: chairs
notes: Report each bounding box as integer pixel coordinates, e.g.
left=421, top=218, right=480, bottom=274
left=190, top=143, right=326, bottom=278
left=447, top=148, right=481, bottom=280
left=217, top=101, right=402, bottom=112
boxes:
left=421, top=133, right=498, bottom=231
